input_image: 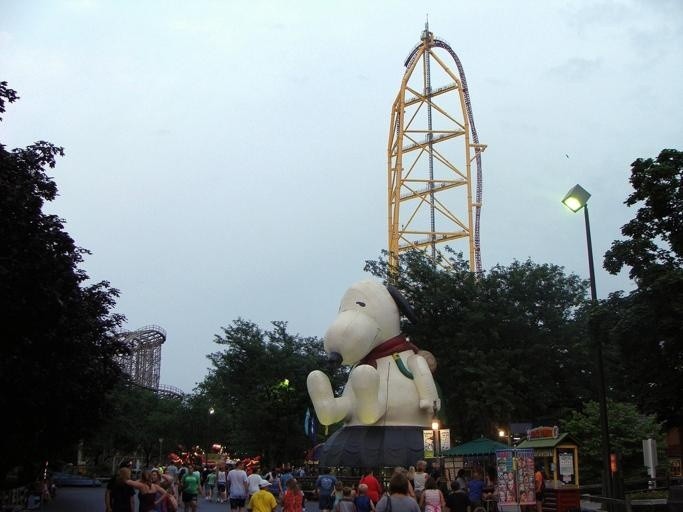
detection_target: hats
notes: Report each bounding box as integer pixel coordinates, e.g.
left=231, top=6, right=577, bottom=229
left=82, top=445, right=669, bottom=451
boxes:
left=259, top=480, right=272, bottom=489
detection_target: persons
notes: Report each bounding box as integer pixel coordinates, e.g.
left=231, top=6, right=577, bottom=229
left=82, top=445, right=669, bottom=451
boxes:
left=535, top=465, right=542, bottom=512
left=105, top=459, right=498, bottom=511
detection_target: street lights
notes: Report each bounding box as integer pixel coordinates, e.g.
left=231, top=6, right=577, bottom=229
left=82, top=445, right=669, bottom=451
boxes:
left=496, top=429, right=522, bottom=448
left=559, top=183, right=615, bottom=511
left=422, top=421, right=451, bottom=458
